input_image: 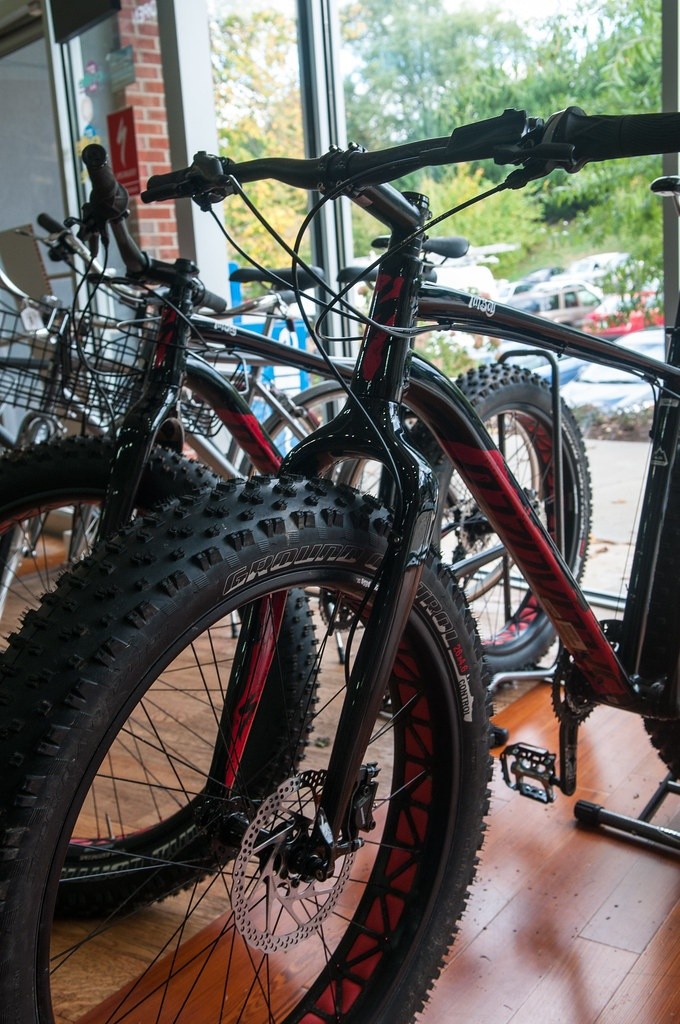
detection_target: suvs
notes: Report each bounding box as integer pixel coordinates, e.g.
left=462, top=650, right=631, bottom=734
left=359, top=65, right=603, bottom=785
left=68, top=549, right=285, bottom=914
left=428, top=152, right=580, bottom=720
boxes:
left=503, top=282, right=605, bottom=330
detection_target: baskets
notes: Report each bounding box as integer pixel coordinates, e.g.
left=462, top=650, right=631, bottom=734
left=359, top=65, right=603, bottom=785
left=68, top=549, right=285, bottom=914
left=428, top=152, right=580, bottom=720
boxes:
left=0, top=283, right=151, bottom=426
left=172, top=341, right=245, bottom=437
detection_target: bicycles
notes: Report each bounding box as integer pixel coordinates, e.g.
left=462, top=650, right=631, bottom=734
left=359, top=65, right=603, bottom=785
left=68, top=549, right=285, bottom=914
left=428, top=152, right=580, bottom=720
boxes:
left=0, top=104, right=679, bottom=1024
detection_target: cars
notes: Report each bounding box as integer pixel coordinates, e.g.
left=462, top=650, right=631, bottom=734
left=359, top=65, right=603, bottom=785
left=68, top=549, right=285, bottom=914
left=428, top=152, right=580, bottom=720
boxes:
left=555, top=330, right=670, bottom=417
left=550, top=251, right=649, bottom=289
